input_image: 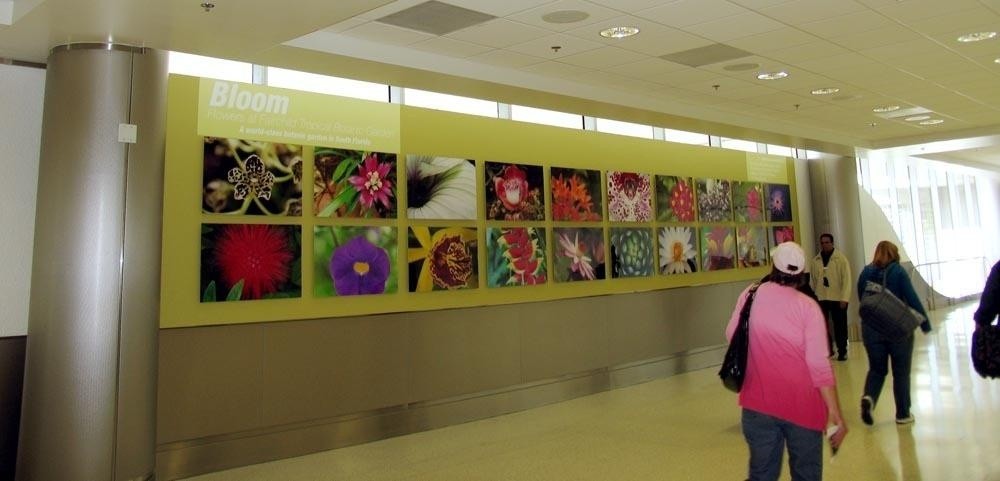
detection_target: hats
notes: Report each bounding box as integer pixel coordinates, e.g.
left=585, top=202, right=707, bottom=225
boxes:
left=770, top=241, right=805, bottom=275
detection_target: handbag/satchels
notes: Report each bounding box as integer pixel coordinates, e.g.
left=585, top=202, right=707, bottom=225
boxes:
left=859, top=279, right=928, bottom=346
left=718, top=312, right=749, bottom=392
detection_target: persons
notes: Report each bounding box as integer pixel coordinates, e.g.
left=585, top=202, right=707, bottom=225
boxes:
left=973, top=260, right=1000, bottom=380
left=725, top=241, right=847, bottom=481
left=857, top=241, right=931, bottom=427
left=808, top=234, right=852, bottom=362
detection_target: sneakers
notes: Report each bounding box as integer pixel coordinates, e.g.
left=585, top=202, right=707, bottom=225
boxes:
left=895, top=412, right=916, bottom=424
left=861, top=395, right=874, bottom=425
left=830, top=352, right=847, bottom=361
